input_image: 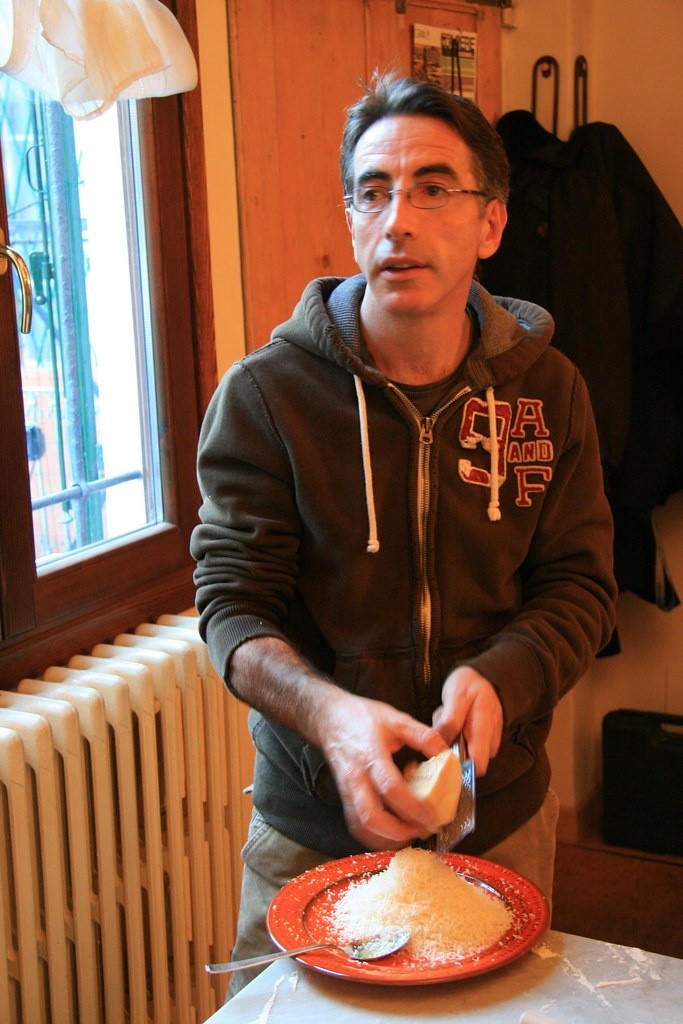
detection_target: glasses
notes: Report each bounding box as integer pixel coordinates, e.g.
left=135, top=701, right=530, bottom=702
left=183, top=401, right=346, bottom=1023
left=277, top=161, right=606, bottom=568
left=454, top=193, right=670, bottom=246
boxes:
left=343, top=181, right=493, bottom=213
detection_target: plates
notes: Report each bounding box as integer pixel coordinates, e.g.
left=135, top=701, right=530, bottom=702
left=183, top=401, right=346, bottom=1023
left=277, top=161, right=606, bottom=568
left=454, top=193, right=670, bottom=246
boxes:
left=266, top=850, right=551, bottom=986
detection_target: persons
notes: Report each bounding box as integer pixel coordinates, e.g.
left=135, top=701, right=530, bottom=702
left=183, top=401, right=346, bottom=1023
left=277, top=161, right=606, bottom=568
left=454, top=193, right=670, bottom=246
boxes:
left=188, top=80, right=619, bottom=1005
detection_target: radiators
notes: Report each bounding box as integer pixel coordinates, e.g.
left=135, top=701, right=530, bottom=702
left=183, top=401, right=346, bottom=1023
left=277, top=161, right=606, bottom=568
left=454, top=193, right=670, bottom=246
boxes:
left=0, top=608, right=253, bottom=1024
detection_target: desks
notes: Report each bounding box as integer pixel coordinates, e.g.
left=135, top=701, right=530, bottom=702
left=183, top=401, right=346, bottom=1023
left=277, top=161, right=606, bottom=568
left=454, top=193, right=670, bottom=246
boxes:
left=199, top=931, right=682, bottom=1023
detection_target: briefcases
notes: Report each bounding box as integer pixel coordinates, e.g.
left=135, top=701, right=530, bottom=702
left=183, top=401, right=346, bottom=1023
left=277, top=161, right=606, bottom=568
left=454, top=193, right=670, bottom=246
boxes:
left=603, top=708, right=683, bottom=856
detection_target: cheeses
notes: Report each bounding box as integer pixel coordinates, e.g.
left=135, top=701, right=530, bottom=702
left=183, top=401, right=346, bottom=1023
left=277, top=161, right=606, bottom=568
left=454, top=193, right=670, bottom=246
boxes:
left=401, top=750, right=462, bottom=826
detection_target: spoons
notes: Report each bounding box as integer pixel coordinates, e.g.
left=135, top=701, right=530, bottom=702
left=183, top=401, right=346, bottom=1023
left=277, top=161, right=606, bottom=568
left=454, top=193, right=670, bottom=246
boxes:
left=205, top=922, right=411, bottom=974
